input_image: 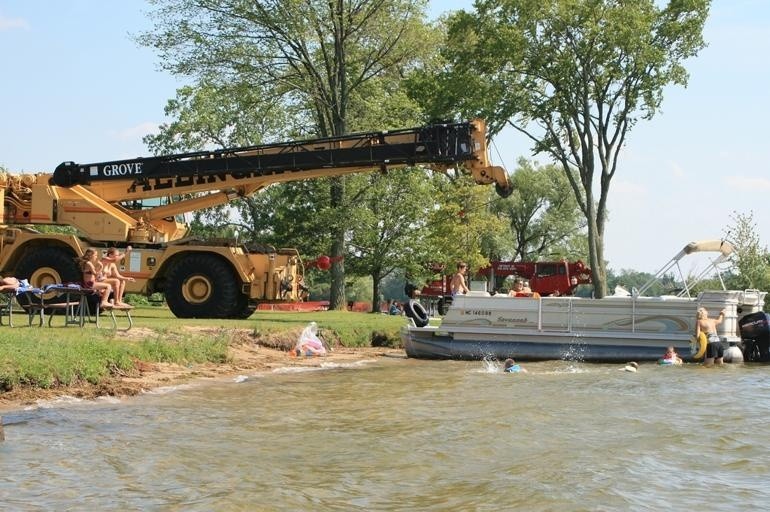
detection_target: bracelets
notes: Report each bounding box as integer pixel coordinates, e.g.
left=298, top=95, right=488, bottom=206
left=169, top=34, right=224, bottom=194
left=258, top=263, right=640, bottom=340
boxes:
left=720, top=311, right=724, bottom=315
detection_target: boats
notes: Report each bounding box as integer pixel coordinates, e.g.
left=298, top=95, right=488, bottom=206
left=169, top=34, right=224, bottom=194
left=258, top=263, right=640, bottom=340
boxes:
left=401, top=238, right=769, bottom=365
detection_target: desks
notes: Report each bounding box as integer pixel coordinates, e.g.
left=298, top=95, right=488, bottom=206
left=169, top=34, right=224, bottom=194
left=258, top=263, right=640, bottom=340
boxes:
left=2, top=284, right=126, bottom=329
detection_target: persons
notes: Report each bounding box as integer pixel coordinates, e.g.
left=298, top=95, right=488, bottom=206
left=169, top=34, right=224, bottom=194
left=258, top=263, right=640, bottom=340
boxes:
left=548, top=290, right=559, bottom=297
left=618, top=361, right=639, bottom=372
left=83, top=245, right=136, bottom=308
left=504, top=358, right=527, bottom=374
left=696, top=308, right=726, bottom=366
left=390, top=301, right=401, bottom=315
left=656, top=346, right=682, bottom=365
left=450, top=262, right=470, bottom=304
left=509, top=279, right=531, bottom=297
left=570, top=275, right=577, bottom=297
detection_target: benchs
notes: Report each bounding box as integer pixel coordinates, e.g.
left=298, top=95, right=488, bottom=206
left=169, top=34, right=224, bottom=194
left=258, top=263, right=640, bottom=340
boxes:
left=97, top=302, right=134, bottom=334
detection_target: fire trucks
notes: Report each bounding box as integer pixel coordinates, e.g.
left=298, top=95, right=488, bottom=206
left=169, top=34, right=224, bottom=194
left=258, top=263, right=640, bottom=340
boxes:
left=405, top=255, right=595, bottom=316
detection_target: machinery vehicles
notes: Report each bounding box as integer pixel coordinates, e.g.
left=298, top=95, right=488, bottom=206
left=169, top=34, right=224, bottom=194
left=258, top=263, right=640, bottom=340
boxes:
left=0, top=118, right=513, bottom=321
